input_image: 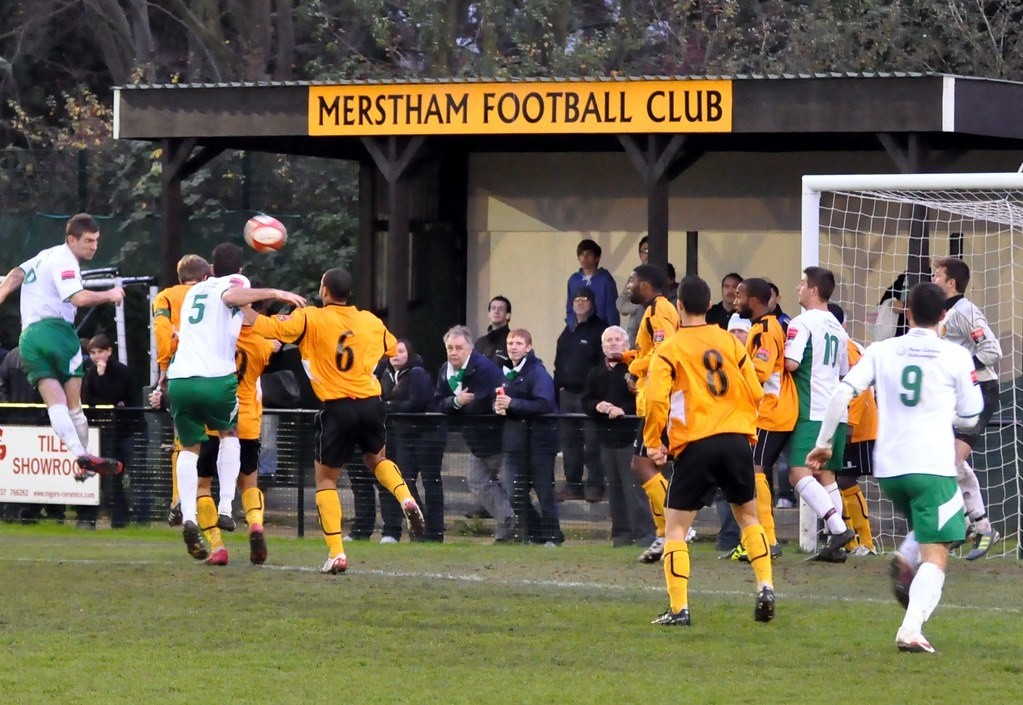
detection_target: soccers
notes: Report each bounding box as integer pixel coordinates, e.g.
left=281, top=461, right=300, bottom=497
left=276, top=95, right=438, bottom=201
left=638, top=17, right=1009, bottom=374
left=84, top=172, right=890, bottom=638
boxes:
left=243, top=215, right=287, bottom=252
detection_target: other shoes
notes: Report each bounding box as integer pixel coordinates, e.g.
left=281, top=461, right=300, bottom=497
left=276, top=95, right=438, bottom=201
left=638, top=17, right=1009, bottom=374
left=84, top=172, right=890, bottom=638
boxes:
left=802, top=548, right=847, bottom=563
left=379, top=536, right=396, bottom=544
left=555, top=484, right=585, bottom=501
left=896, top=627, right=935, bottom=652
left=638, top=537, right=665, bottom=564
left=887, top=551, right=916, bottom=609
left=586, top=486, right=603, bottom=502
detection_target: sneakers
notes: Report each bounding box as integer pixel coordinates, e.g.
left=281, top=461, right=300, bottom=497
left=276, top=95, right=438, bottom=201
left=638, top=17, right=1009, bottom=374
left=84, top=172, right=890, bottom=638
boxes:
left=717, top=543, right=749, bottom=561
left=816, top=524, right=855, bottom=550
left=74, top=468, right=96, bottom=483
left=216, top=514, right=236, bottom=532
left=965, top=528, right=1000, bottom=561
left=77, top=453, right=123, bottom=476
left=650, top=608, right=690, bottom=626
left=183, top=520, right=209, bottom=561
left=754, top=586, right=775, bottom=623
left=950, top=524, right=976, bottom=552
left=400, top=497, right=425, bottom=536
left=168, top=501, right=183, bottom=528
left=204, top=549, right=228, bottom=565
left=250, top=523, right=267, bottom=564
left=320, top=554, right=347, bottom=574
left=770, top=543, right=782, bottom=560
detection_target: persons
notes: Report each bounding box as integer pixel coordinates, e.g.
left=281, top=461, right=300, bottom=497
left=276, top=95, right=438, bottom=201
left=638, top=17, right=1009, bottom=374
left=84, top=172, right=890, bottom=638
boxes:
left=875, top=259, right=1003, bottom=561
left=238, top=268, right=425, bottom=574
left=260, top=296, right=565, bottom=547
left=551, top=235, right=879, bottom=565
left=0, top=334, right=135, bottom=531
left=642, top=274, right=777, bottom=627
left=805, top=283, right=979, bottom=653
left=148, top=242, right=308, bottom=566
left=0, top=214, right=125, bottom=481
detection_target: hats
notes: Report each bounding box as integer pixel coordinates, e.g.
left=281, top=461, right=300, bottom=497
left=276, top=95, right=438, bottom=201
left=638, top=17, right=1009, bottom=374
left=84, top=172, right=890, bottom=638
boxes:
left=572, top=286, right=596, bottom=304
